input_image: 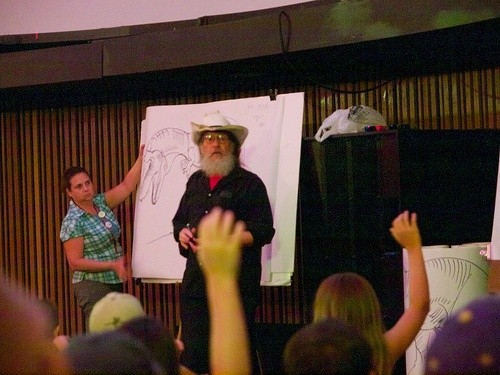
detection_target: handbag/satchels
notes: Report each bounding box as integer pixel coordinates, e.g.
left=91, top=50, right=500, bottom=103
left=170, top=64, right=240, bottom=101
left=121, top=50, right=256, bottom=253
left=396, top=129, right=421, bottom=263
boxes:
left=315, top=105, right=387, bottom=143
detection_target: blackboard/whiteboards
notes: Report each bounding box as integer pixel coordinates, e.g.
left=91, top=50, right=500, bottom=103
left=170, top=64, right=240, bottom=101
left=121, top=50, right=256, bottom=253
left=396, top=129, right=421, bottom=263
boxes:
left=294, top=128, right=500, bottom=333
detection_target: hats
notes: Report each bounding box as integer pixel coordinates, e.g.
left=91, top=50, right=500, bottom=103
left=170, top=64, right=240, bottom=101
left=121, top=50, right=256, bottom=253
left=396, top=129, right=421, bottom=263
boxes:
left=425, top=298, right=500, bottom=375
left=90, top=291, right=143, bottom=333
left=191, top=111, right=249, bottom=146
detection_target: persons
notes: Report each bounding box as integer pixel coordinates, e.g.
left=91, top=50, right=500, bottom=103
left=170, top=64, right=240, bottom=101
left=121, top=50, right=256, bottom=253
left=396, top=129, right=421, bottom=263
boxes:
left=60, top=144, right=144, bottom=335
left=314, top=211, right=431, bottom=375
left=0, top=207, right=374, bottom=375
left=173, top=114, right=276, bottom=375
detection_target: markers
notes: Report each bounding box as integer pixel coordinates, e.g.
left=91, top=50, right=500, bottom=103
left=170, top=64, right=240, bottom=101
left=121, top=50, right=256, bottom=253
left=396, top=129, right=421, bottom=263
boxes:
left=187, top=223, right=192, bottom=242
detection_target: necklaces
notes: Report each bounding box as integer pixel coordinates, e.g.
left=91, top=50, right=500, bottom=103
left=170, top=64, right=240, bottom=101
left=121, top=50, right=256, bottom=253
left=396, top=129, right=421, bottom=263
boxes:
left=75, top=204, right=117, bottom=254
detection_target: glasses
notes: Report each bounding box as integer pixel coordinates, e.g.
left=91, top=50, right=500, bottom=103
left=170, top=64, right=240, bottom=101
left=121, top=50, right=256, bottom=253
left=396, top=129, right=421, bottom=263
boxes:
left=202, top=133, right=229, bottom=144
